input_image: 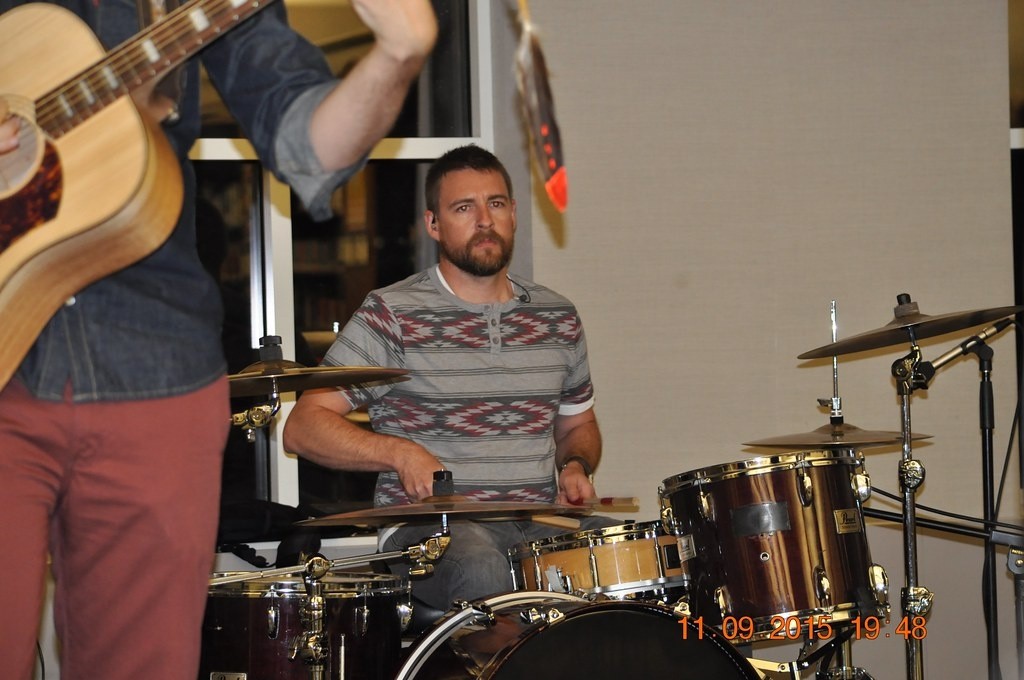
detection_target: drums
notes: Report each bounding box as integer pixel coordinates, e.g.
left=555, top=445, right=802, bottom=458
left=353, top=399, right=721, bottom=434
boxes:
left=394, top=590, right=761, bottom=680
left=506, top=517, right=686, bottom=602
left=658, top=448, right=892, bottom=647
left=198, top=570, right=403, bottom=680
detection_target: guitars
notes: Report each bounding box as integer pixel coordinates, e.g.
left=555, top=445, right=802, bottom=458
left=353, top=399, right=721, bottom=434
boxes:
left=0, top=0, right=278, bottom=399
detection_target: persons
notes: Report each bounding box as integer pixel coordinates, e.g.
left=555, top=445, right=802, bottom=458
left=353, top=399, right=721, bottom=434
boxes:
left=282, top=145, right=602, bottom=612
left=0, top=0, right=440, bottom=679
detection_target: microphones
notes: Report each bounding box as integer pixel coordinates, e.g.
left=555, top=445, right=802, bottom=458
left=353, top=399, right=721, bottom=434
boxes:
left=505, top=275, right=532, bottom=304
left=431, top=469, right=457, bottom=535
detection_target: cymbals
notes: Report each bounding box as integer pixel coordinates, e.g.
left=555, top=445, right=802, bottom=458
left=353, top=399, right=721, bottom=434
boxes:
left=797, top=303, right=1019, bottom=361
left=741, top=419, right=936, bottom=451
left=290, top=494, right=595, bottom=527
left=226, top=360, right=412, bottom=399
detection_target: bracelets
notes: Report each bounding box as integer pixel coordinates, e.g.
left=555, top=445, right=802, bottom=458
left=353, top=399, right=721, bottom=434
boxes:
left=558, top=456, right=594, bottom=485
